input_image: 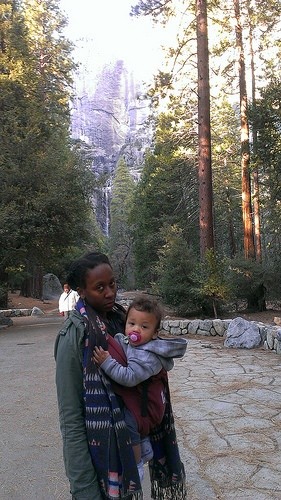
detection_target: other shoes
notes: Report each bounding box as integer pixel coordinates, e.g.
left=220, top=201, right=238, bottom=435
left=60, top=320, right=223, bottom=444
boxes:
left=140, top=438, right=154, bottom=461
left=137, top=462, right=145, bottom=482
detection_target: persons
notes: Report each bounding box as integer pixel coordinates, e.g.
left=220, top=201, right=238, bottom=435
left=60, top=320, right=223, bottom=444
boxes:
left=94, top=297, right=188, bottom=482
left=59, top=284, right=80, bottom=319
left=54, top=251, right=128, bottom=500
